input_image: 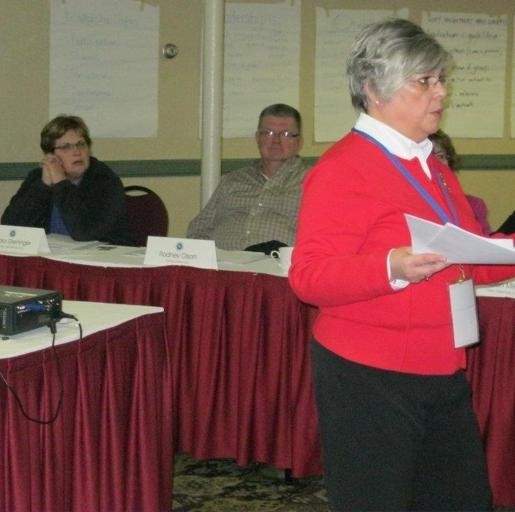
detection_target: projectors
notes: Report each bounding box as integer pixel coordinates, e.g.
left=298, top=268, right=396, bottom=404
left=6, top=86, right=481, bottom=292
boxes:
left=0, top=284, right=62, bottom=336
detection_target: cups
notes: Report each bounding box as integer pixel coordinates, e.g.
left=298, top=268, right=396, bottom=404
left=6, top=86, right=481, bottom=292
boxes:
left=270, top=246, right=294, bottom=272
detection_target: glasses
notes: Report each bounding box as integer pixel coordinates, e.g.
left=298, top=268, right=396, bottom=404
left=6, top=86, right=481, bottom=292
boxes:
left=55, top=140, right=88, bottom=151
left=257, top=129, right=299, bottom=139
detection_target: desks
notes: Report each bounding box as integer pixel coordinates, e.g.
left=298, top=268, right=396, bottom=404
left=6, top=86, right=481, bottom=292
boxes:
left=2, top=232, right=512, bottom=507
left=0, top=296, right=177, bottom=509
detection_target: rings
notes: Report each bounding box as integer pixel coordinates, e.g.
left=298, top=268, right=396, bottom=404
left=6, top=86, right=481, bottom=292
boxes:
left=425, top=271, right=428, bottom=280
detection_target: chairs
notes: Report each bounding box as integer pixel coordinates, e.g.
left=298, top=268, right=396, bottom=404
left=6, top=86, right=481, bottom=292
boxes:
left=120, top=186, right=168, bottom=246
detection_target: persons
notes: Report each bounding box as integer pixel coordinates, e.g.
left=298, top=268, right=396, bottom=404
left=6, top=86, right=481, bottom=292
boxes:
left=428, top=129, right=491, bottom=234
left=287, top=17, right=515, bottom=512
left=1, top=115, right=139, bottom=246
left=185, top=104, right=314, bottom=250
